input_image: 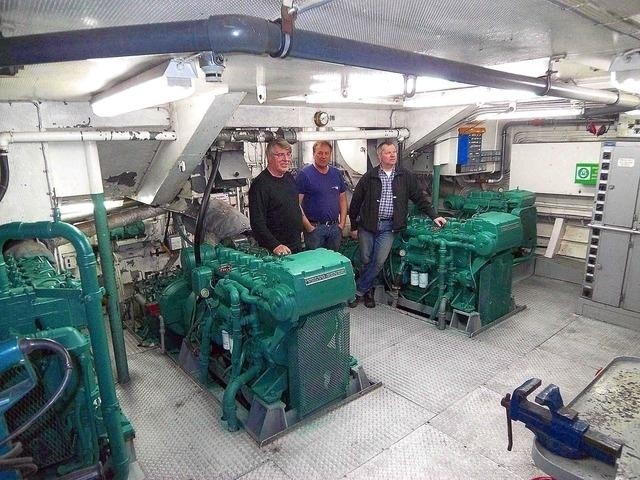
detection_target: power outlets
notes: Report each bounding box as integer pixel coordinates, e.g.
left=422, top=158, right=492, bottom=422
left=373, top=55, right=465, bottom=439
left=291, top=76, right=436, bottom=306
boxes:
left=509, top=102, right=516, bottom=111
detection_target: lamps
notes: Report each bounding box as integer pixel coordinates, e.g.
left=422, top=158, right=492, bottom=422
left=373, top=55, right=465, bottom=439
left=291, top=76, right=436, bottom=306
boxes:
left=88, top=59, right=198, bottom=117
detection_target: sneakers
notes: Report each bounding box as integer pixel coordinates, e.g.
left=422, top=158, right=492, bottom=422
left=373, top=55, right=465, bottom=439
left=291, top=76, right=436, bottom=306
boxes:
left=350, top=285, right=376, bottom=309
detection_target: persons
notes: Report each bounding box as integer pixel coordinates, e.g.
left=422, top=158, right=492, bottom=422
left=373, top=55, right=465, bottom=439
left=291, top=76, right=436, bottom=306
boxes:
left=247, top=138, right=304, bottom=257
left=294, top=140, right=349, bottom=252
left=348, top=140, right=448, bottom=309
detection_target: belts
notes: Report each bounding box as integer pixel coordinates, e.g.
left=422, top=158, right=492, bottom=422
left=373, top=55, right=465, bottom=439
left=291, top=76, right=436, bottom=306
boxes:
left=309, top=218, right=337, bottom=225
left=377, top=217, right=391, bottom=221
